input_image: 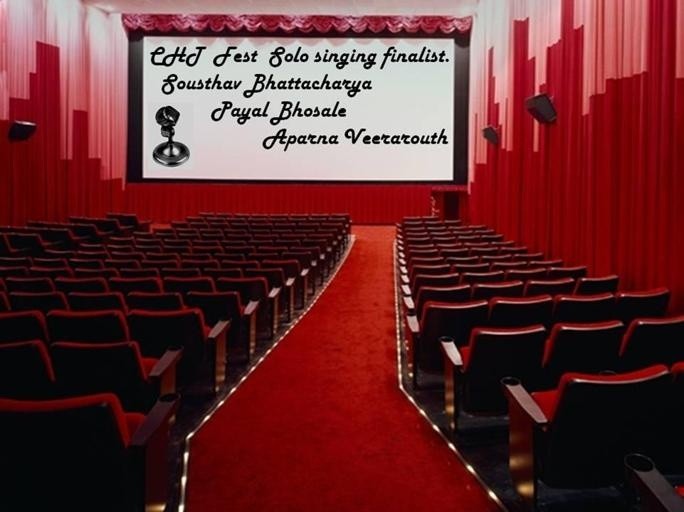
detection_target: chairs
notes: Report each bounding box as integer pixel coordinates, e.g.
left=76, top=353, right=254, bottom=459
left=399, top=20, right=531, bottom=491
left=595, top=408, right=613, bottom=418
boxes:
left=392, top=216, right=683, bottom=512
left=0, top=213, right=353, bottom=512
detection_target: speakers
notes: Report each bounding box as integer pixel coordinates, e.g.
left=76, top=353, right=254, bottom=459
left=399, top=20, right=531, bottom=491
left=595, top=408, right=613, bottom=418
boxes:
left=8, top=119, right=36, bottom=141
left=482, top=127, right=499, bottom=145
left=525, top=94, right=556, bottom=125
